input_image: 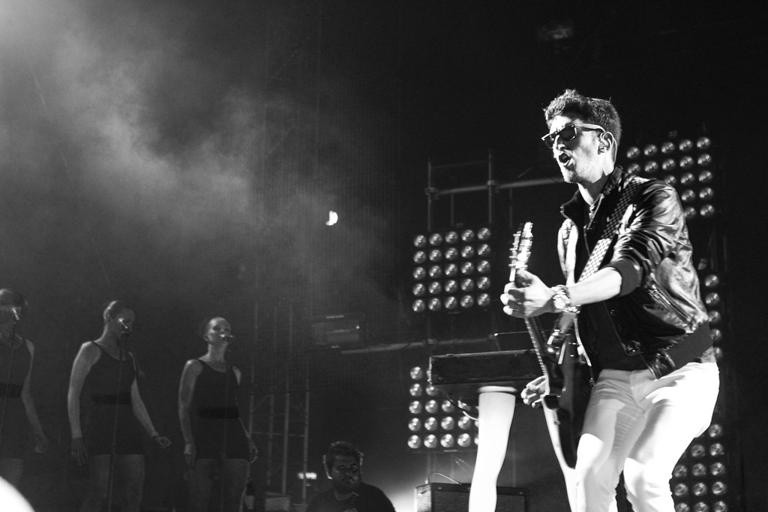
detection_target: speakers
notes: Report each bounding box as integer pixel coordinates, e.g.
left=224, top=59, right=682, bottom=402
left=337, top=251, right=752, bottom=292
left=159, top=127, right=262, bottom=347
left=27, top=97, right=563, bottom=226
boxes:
left=416, top=482, right=527, bottom=512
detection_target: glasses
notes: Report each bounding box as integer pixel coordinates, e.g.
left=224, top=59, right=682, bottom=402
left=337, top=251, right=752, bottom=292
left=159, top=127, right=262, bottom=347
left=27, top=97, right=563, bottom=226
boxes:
left=540, top=122, right=605, bottom=150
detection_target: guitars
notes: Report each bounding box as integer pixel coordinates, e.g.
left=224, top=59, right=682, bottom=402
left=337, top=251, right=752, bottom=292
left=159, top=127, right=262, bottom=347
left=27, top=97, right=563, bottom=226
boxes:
left=508, top=221, right=590, bottom=467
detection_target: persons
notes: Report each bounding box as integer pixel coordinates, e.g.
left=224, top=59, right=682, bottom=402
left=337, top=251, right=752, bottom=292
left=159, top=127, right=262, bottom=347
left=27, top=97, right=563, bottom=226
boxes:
left=177, top=316, right=261, bottom=511
left=68, top=296, right=171, bottom=511
left=499, top=84, right=722, bottom=511
left=307, top=441, right=396, bottom=512
left=0, top=286, right=49, bottom=511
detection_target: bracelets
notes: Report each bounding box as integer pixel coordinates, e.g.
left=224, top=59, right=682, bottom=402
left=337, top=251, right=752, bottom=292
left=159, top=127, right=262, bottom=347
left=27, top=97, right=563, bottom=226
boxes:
left=558, top=284, right=579, bottom=314
left=183, top=439, right=194, bottom=445
left=151, top=435, right=160, bottom=438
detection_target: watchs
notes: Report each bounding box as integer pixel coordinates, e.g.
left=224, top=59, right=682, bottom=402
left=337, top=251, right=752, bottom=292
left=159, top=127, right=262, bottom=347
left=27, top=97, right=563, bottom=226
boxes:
left=550, top=283, right=567, bottom=315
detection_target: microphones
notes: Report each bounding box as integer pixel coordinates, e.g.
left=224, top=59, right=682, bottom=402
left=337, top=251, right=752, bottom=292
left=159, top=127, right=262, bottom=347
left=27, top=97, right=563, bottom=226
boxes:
left=219, top=334, right=236, bottom=342
left=8, top=304, right=20, bottom=321
left=117, top=317, right=129, bottom=331
